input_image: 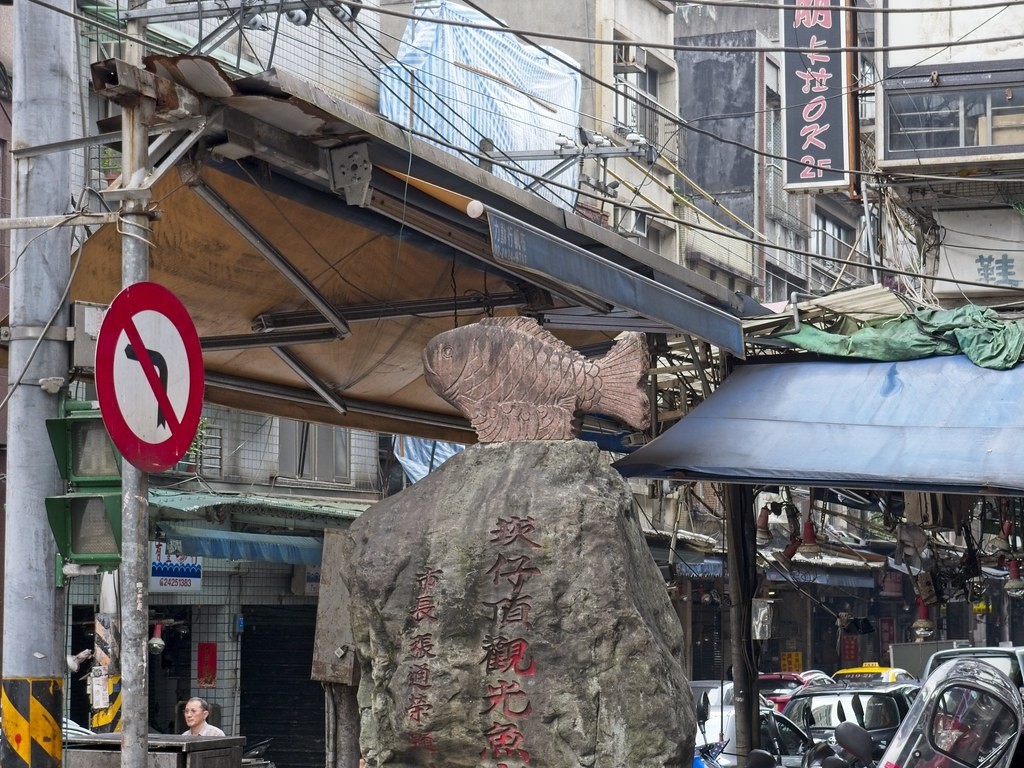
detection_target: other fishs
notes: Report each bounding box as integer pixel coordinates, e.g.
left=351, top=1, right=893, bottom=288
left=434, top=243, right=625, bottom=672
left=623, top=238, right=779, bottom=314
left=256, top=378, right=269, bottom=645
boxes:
left=420, top=315, right=653, bottom=443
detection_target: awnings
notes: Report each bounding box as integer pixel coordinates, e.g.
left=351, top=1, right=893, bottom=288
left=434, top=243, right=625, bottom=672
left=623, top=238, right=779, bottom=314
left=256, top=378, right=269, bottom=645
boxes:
left=612, top=351, right=1024, bottom=500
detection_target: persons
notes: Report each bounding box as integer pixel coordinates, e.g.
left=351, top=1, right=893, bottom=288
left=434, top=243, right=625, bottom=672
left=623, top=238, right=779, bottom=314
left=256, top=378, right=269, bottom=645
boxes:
left=182, top=697, right=226, bottom=737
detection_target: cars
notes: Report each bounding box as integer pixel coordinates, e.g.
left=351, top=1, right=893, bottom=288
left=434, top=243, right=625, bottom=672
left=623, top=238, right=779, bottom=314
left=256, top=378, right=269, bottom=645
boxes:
left=686, top=643, right=1024, bottom=768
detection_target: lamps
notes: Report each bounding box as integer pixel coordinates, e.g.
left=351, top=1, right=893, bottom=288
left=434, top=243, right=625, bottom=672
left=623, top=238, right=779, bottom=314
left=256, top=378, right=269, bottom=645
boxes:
left=752, top=482, right=1024, bottom=635
left=147, top=624, right=166, bottom=655
